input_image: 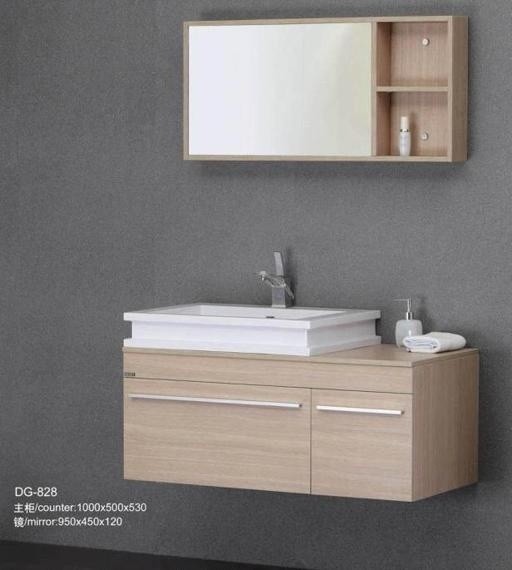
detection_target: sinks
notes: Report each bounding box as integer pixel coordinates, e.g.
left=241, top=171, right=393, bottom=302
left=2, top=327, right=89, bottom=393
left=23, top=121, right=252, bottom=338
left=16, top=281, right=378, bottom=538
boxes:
left=123, top=301, right=383, bottom=356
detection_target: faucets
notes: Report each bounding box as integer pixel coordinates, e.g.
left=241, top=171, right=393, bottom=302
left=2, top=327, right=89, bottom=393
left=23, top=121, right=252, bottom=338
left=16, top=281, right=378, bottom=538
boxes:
left=259, top=251, right=296, bottom=309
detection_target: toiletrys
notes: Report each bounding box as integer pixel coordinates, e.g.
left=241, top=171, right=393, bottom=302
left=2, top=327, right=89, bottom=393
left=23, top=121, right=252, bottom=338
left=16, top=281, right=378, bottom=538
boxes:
left=399, top=116, right=411, bottom=156
